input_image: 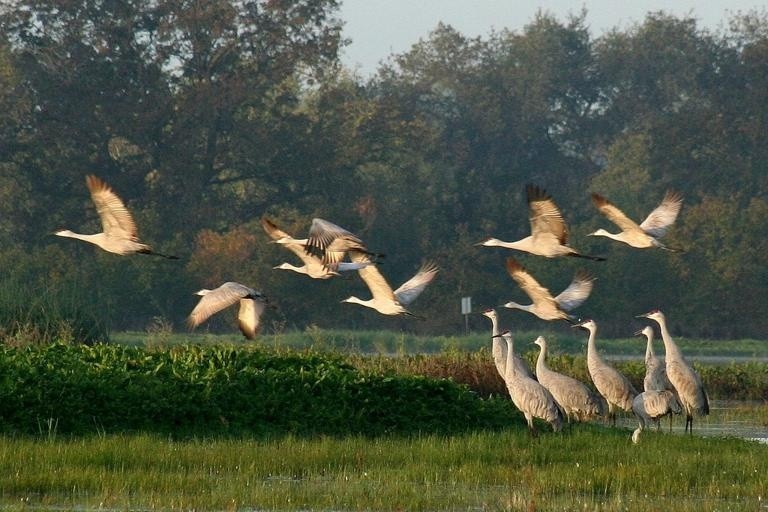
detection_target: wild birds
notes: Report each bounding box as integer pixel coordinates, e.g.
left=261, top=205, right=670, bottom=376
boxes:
left=498, top=256, right=597, bottom=328
left=187, top=281, right=272, bottom=339
left=587, top=186, right=692, bottom=255
left=480, top=306, right=712, bottom=445
left=256, top=216, right=390, bottom=281
left=49, top=174, right=179, bottom=260
left=340, top=248, right=443, bottom=321
left=471, top=182, right=607, bottom=262
